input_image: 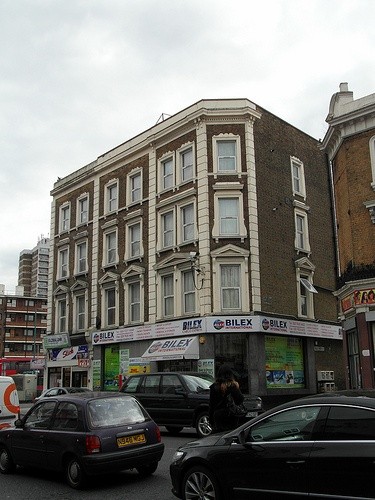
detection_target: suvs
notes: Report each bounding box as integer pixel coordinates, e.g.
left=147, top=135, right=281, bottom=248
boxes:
left=116, top=372, right=263, bottom=438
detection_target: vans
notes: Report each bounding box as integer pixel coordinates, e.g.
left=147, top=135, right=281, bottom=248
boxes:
left=0, top=376, right=20, bottom=429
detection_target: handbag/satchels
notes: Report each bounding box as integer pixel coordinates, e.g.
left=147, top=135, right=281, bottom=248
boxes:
left=228, top=394, right=247, bottom=418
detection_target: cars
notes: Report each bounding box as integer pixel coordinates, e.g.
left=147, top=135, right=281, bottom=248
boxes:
left=33, top=387, right=95, bottom=412
left=166, top=393, right=374, bottom=499
left=0, top=391, right=165, bottom=489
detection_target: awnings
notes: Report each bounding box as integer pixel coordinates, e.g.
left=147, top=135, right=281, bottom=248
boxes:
left=140, top=335, right=200, bottom=361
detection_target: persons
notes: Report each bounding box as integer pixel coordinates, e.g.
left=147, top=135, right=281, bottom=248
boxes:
left=208, top=364, right=243, bottom=431
